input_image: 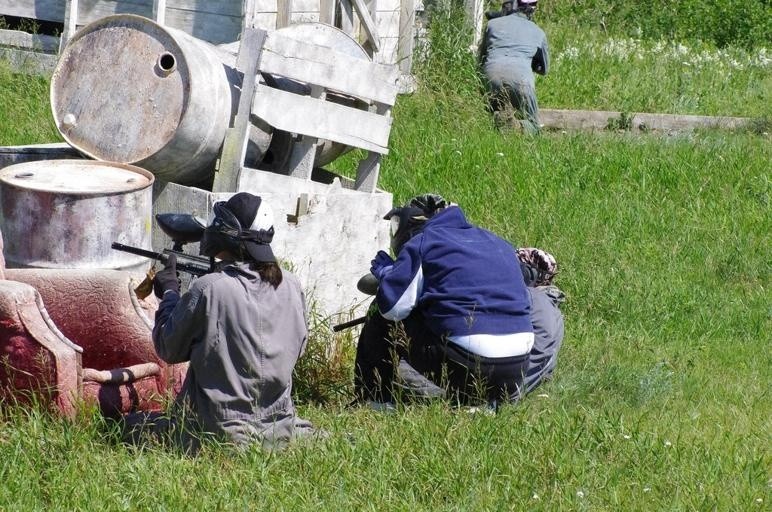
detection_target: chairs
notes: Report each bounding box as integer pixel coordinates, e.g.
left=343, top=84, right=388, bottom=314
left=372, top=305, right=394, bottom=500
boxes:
left=0, top=228, right=189, bottom=417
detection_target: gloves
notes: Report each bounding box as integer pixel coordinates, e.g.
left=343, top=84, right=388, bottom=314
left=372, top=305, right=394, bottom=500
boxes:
left=370, top=249, right=396, bottom=278
left=154, top=252, right=182, bottom=297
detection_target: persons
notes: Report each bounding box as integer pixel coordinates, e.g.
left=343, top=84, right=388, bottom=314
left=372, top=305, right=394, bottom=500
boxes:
left=149, top=190, right=317, bottom=457
left=342, top=191, right=536, bottom=410
left=474, top=0, right=551, bottom=137
left=512, top=245, right=570, bottom=398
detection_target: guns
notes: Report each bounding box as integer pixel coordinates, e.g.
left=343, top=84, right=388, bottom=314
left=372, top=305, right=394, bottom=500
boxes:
left=111, top=214, right=218, bottom=278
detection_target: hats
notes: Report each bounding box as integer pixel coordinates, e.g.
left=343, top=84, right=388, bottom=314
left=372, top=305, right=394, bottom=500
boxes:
left=389, top=192, right=447, bottom=244
left=218, top=191, right=277, bottom=266
left=514, top=246, right=557, bottom=288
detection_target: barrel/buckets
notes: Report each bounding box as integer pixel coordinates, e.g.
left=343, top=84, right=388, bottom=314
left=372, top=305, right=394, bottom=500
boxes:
left=50, top=13, right=239, bottom=183
left=21, top=141, right=80, bottom=161
left=0, top=160, right=155, bottom=298
